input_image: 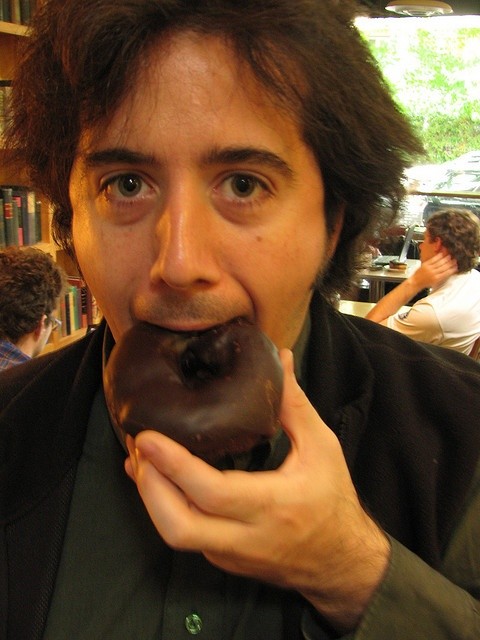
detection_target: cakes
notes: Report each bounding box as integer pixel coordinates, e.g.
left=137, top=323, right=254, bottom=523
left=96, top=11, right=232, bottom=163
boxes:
left=389, top=259, right=407, bottom=269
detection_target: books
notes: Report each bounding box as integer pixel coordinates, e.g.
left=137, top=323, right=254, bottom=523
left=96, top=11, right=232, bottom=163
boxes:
left=0, top=181, right=67, bottom=247
left=0, top=0, right=41, bottom=30
left=1, top=84, right=25, bottom=146
left=59, top=274, right=104, bottom=337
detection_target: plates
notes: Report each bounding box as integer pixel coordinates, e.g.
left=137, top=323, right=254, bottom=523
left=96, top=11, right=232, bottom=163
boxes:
left=384, top=265, right=411, bottom=272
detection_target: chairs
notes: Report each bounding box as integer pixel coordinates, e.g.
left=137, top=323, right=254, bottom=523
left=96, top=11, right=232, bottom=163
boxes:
left=467, top=337, right=480, bottom=360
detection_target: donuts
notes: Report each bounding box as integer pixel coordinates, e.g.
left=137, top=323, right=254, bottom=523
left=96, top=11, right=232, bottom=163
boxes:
left=102, top=316, right=284, bottom=465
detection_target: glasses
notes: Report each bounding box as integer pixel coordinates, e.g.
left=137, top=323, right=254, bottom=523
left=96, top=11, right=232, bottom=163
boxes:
left=48, top=316, right=62, bottom=330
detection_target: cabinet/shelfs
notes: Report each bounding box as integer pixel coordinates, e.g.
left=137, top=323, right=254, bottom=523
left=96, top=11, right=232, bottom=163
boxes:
left=1, top=2, right=114, bottom=358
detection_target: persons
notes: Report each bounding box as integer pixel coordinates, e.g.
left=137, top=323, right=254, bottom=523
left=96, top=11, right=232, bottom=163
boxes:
left=0, top=244, right=65, bottom=372
left=0, top=0, right=479, bottom=635
left=362, top=203, right=480, bottom=360
